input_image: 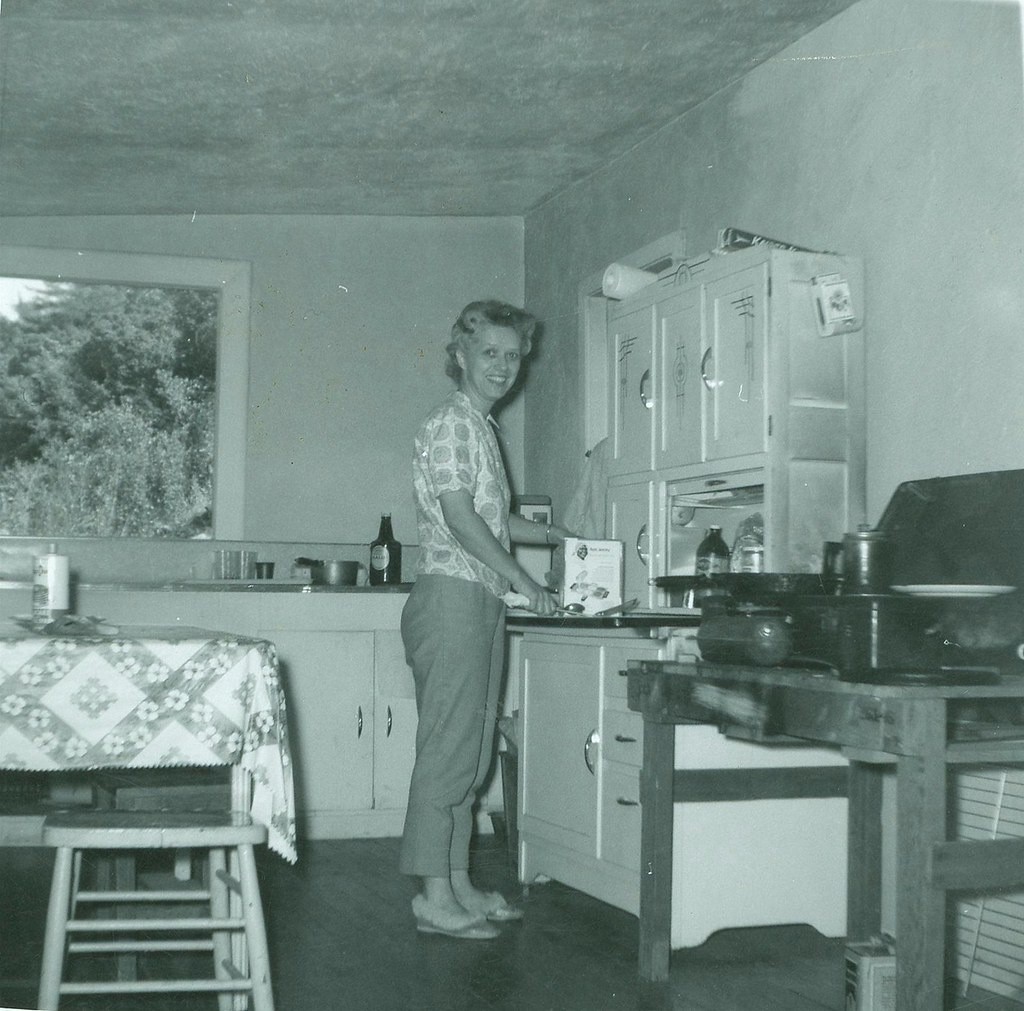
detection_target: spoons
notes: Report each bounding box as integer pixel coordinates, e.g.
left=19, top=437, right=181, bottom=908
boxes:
left=557, top=603, right=585, bottom=616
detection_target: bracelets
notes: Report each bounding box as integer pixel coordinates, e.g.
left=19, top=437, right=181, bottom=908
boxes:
left=546, top=524, right=552, bottom=543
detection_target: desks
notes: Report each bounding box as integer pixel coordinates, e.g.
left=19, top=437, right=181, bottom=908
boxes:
left=0, top=624, right=297, bottom=1011
left=627, top=656, right=1024, bottom=1011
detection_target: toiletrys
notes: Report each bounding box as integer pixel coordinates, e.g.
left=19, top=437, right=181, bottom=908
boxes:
left=30, top=542, right=71, bottom=632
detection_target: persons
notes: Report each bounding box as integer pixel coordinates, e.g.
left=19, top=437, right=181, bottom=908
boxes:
left=399, top=301, right=581, bottom=940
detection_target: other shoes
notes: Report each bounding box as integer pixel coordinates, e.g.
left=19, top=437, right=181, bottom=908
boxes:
left=411, top=892, right=499, bottom=940
left=457, top=892, right=523, bottom=922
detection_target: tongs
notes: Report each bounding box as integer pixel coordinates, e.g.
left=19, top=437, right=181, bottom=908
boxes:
left=594, top=598, right=641, bottom=616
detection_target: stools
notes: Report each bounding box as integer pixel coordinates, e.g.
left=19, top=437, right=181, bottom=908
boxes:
left=39, top=811, right=276, bottom=1011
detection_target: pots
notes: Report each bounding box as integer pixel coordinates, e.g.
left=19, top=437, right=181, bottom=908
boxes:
left=295, top=557, right=358, bottom=586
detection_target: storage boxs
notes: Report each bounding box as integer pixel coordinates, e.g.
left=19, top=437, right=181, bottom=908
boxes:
left=553, top=537, right=625, bottom=616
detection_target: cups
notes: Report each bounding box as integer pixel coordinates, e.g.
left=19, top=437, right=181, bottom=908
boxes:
left=215, top=551, right=240, bottom=579
left=819, top=524, right=893, bottom=595
left=255, top=562, right=274, bottom=580
left=241, top=552, right=259, bottom=579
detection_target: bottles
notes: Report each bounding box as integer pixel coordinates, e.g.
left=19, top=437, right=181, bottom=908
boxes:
left=730, top=524, right=764, bottom=573
left=370, top=512, right=402, bottom=585
left=31, top=544, right=70, bottom=632
left=693, top=524, right=731, bottom=609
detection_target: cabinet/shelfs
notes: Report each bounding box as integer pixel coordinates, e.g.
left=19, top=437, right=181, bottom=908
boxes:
left=520, top=246, right=863, bottom=954
left=262, top=628, right=515, bottom=816
